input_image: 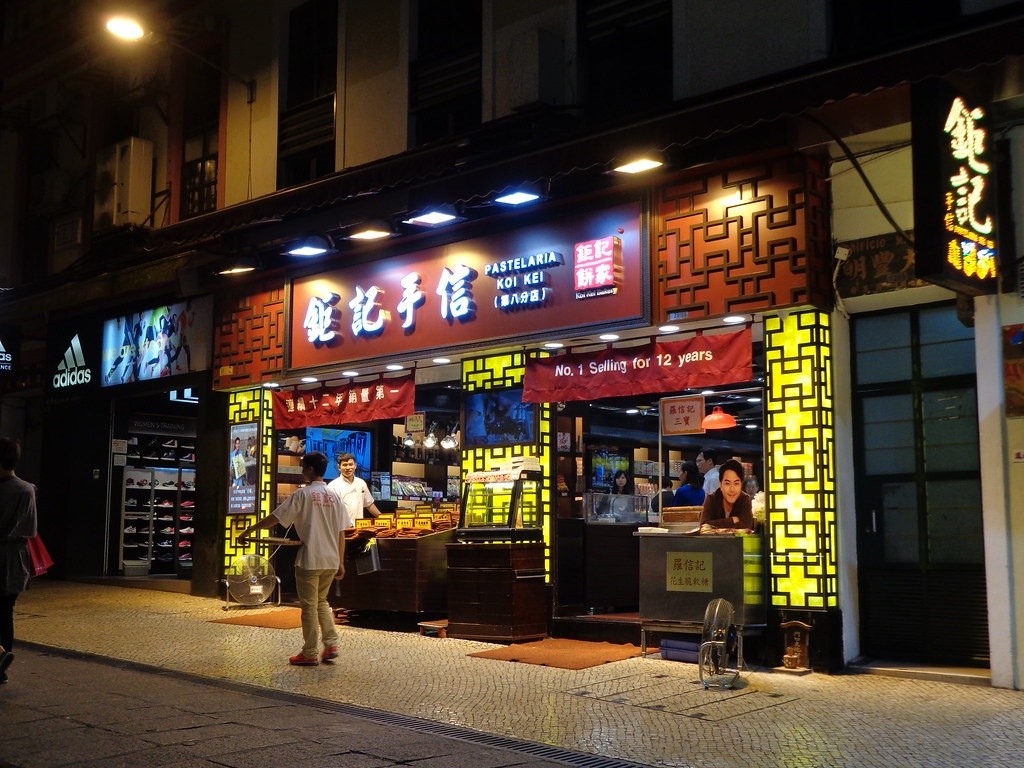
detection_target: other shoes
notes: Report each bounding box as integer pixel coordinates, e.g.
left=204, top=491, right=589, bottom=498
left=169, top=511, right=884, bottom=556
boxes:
left=0, top=673, right=8, bottom=683
left=0, top=651, right=14, bottom=672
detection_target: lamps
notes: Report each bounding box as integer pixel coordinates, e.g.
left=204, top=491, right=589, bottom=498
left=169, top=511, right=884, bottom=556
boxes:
left=702, top=396, right=737, bottom=429
left=403, top=414, right=458, bottom=449
left=94, top=6, right=258, bottom=103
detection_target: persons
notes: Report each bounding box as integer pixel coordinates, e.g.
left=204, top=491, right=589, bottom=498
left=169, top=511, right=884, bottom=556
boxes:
left=0, top=438, right=37, bottom=683
left=230, top=434, right=258, bottom=486
left=327, top=453, right=382, bottom=619
left=669, top=462, right=705, bottom=507
left=102, top=301, right=194, bottom=386
left=700, top=459, right=753, bottom=530
left=650, top=474, right=673, bottom=512
left=604, top=470, right=635, bottom=514
left=697, top=448, right=722, bottom=507
left=239, top=452, right=348, bottom=666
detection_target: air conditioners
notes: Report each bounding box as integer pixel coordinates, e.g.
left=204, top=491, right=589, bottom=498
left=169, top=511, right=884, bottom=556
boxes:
left=90, top=137, right=153, bottom=233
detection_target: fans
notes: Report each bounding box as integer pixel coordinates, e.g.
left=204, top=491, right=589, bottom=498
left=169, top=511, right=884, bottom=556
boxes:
left=699, top=597, right=744, bottom=691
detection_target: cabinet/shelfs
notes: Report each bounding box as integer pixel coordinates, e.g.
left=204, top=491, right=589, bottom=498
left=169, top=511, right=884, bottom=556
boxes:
left=118, top=443, right=197, bottom=570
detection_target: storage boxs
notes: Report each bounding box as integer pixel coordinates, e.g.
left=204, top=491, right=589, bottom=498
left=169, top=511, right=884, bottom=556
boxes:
left=660, top=637, right=702, bottom=664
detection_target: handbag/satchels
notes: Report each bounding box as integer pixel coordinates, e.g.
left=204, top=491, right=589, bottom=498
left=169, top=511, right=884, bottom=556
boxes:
left=20, top=532, right=53, bottom=579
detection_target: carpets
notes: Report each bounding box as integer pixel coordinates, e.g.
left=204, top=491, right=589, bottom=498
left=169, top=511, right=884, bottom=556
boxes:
left=205, top=608, right=298, bottom=630
left=467, top=638, right=661, bottom=671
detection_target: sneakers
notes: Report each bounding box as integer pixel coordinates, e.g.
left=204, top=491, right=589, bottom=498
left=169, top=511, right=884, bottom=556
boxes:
left=123, top=479, right=195, bottom=560
left=319, top=645, right=338, bottom=659
left=289, top=652, right=319, bottom=666
left=126, top=437, right=196, bottom=462
